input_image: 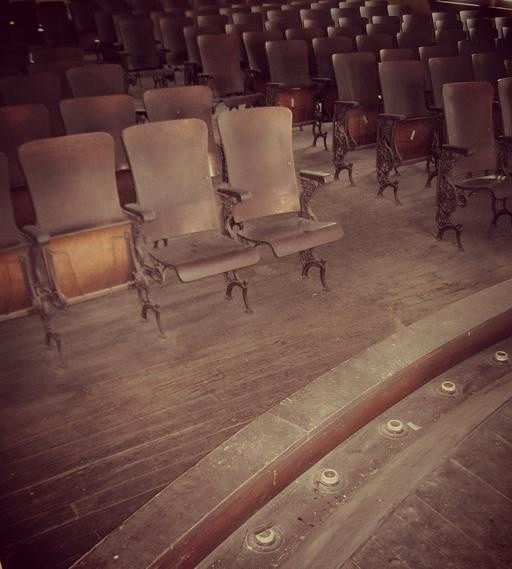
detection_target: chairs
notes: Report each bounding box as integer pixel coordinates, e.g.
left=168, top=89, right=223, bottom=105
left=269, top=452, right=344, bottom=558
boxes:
left=2, top=3, right=510, bottom=206
left=429, top=78, right=510, bottom=252
left=120, top=118, right=260, bottom=341
left=16, top=129, right=167, bottom=372
left=218, top=106, right=345, bottom=293
left=2, top=155, right=69, bottom=370
